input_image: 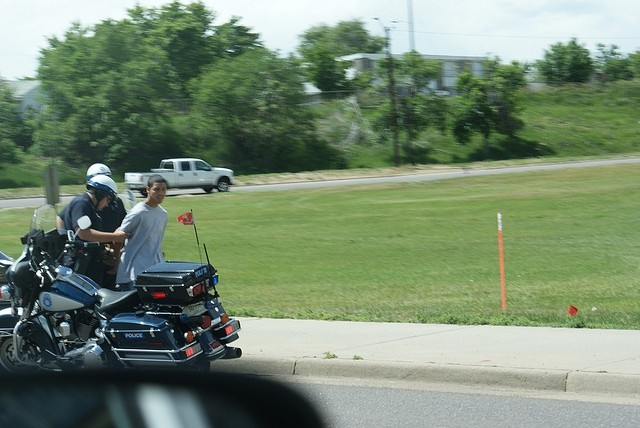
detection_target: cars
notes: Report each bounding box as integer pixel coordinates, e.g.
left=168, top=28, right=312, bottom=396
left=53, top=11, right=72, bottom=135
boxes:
left=0, top=250, right=17, bottom=310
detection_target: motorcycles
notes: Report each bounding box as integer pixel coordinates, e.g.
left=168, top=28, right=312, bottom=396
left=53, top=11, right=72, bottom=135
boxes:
left=0, top=204, right=241, bottom=374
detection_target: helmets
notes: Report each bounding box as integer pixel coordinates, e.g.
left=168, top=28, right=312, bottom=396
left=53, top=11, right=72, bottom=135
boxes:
left=87, top=163, right=112, bottom=180
left=86, top=175, right=118, bottom=200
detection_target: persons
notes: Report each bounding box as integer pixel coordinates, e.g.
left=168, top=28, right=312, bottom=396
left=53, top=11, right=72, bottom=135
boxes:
left=114, top=174, right=168, bottom=291
left=86, top=162, right=127, bottom=255
left=56, top=174, right=132, bottom=280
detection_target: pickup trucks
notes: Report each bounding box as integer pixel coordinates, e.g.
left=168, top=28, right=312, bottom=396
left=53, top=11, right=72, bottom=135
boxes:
left=125, top=158, right=234, bottom=196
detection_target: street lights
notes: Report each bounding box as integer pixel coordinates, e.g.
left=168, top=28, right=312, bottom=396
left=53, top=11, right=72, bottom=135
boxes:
left=373, top=14, right=405, bottom=168
